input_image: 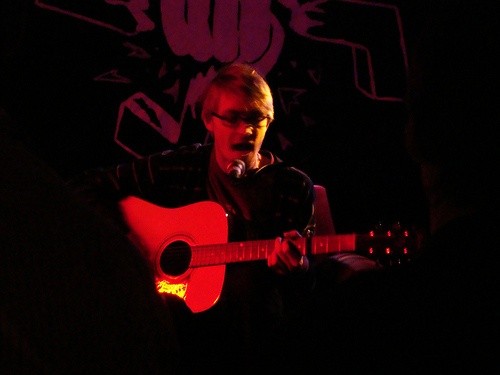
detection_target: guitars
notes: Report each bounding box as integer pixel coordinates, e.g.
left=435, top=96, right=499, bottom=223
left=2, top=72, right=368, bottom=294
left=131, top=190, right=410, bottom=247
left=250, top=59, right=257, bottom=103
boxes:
left=114, top=193, right=416, bottom=320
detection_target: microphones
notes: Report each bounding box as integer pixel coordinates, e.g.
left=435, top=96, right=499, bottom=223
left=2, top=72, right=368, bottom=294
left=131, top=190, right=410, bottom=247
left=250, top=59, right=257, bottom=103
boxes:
left=226, top=158, right=246, bottom=180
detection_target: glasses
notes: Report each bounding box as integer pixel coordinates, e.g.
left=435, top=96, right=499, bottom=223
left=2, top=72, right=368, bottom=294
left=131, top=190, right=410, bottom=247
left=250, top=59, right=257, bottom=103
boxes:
left=211, top=111, right=272, bottom=127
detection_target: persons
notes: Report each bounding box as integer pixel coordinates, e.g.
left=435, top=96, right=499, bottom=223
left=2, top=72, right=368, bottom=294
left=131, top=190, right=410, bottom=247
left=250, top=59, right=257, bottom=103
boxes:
left=150, top=61, right=317, bottom=280
